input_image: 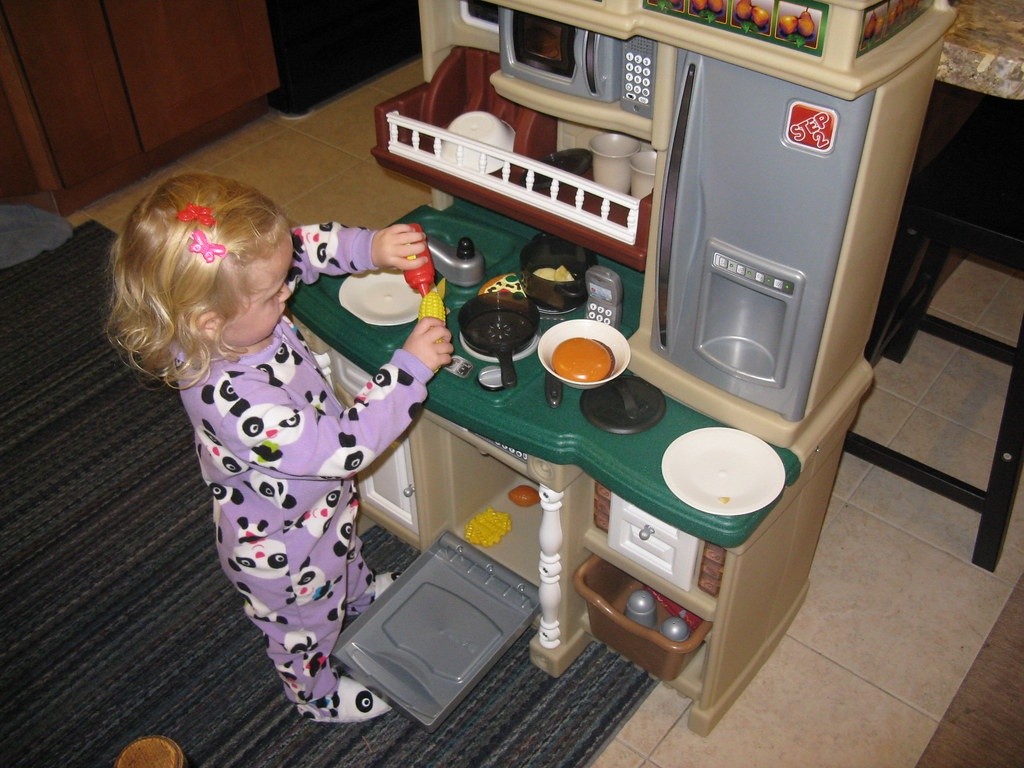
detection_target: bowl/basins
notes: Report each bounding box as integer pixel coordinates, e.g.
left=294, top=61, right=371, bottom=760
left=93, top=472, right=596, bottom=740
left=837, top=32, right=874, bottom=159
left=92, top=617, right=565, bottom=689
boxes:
left=537, top=318, right=633, bottom=389
left=435, top=109, right=517, bottom=174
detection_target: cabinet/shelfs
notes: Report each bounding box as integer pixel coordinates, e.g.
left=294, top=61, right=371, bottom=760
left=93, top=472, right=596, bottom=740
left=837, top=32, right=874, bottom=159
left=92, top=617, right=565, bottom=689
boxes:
left=845, top=99, right=1024, bottom=573
left=0, top=0, right=281, bottom=216
left=287, top=315, right=421, bottom=549
left=577, top=475, right=737, bottom=710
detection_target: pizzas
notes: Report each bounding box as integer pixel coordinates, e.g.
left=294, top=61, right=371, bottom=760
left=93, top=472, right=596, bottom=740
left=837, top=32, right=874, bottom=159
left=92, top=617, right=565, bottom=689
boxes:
left=478, top=272, right=527, bottom=297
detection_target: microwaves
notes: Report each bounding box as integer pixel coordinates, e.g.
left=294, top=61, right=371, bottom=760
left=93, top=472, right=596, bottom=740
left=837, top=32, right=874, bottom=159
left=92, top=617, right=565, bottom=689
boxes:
left=496, top=5, right=625, bottom=104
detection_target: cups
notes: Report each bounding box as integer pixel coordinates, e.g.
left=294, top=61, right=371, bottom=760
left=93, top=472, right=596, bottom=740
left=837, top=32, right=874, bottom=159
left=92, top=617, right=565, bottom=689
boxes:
left=589, top=131, right=641, bottom=197
left=629, top=150, right=660, bottom=198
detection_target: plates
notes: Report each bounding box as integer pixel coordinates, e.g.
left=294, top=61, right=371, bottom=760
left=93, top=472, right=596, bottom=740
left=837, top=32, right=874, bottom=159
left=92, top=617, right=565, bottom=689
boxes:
left=661, top=426, right=786, bottom=516
left=339, top=265, right=434, bottom=326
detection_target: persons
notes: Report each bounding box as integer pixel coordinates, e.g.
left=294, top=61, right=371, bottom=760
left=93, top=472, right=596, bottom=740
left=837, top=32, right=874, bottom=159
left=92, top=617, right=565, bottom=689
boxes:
left=104, top=172, right=454, bottom=723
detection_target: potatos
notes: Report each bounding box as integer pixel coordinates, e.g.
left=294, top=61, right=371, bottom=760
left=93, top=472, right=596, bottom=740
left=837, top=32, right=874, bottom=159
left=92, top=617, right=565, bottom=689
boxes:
left=507, top=485, right=540, bottom=507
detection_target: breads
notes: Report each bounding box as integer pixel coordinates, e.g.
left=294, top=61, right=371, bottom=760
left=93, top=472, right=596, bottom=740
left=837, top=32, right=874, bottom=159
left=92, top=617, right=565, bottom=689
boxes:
left=552, top=336, right=615, bottom=383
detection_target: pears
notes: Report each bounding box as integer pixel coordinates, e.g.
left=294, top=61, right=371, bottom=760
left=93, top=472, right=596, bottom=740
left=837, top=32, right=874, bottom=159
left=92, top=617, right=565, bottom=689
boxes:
left=864, top=0, right=920, bottom=39
left=669, top=0, right=816, bottom=38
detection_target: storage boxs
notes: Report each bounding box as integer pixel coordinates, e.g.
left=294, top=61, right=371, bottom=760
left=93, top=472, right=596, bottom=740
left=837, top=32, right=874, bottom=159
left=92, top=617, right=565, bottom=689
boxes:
left=572, top=554, right=713, bottom=681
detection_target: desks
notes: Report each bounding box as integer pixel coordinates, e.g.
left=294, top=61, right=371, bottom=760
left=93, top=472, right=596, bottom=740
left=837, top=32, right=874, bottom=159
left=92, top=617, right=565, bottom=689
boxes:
left=935, top=0, right=1024, bottom=100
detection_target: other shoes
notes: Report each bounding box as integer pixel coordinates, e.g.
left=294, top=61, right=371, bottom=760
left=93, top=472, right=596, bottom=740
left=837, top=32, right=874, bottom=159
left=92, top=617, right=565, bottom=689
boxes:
left=346, top=571, right=402, bottom=615
left=296, top=676, right=393, bottom=722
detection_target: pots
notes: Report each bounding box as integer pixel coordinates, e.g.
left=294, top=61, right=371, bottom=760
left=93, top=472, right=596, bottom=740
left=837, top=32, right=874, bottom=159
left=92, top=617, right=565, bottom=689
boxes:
left=458, top=289, right=541, bottom=388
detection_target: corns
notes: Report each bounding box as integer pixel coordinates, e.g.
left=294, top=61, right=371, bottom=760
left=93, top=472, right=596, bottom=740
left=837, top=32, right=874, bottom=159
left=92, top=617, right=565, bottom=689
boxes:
left=418, top=291, right=446, bottom=345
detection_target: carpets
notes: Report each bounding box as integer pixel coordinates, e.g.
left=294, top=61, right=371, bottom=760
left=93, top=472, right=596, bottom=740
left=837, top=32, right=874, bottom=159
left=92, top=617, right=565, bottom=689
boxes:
left=916, top=573, right=1024, bottom=768
left=0, top=219, right=655, bottom=768
